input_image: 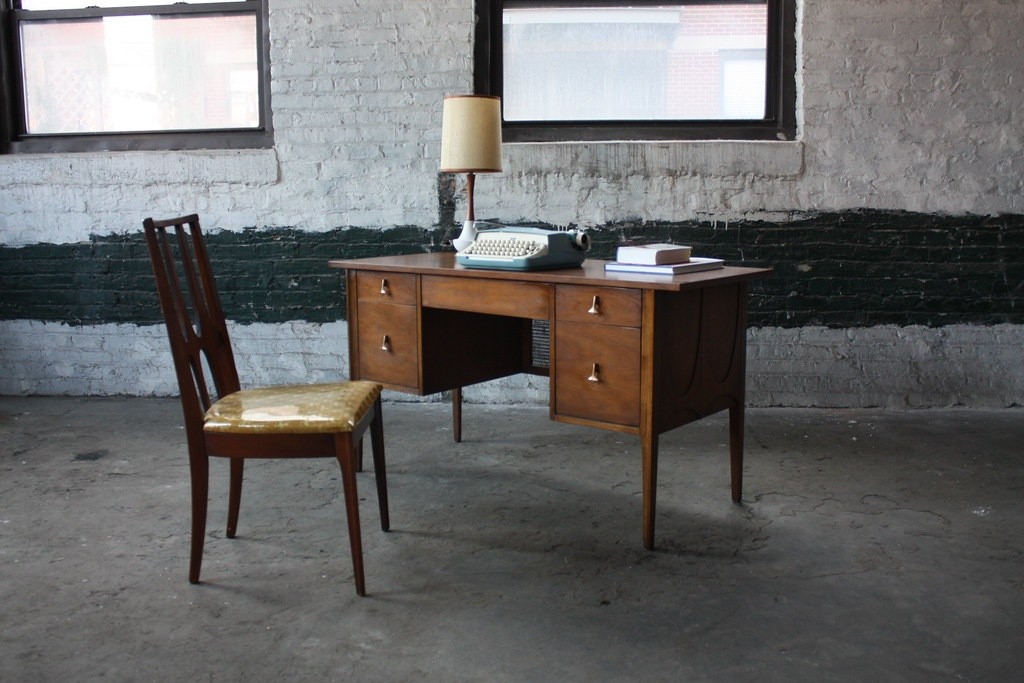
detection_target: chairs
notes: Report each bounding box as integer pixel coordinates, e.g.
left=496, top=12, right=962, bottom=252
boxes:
left=142, top=213, right=392, bottom=596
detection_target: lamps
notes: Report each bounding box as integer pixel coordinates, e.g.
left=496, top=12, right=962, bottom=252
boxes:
left=439, top=94, right=502, bottom=253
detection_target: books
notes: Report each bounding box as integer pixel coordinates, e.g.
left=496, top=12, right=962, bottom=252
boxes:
left=604, top=243, right=725, bottom=275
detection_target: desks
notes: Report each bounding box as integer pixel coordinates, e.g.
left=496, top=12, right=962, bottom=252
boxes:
left=327, top=252, right=772, bottom=551
